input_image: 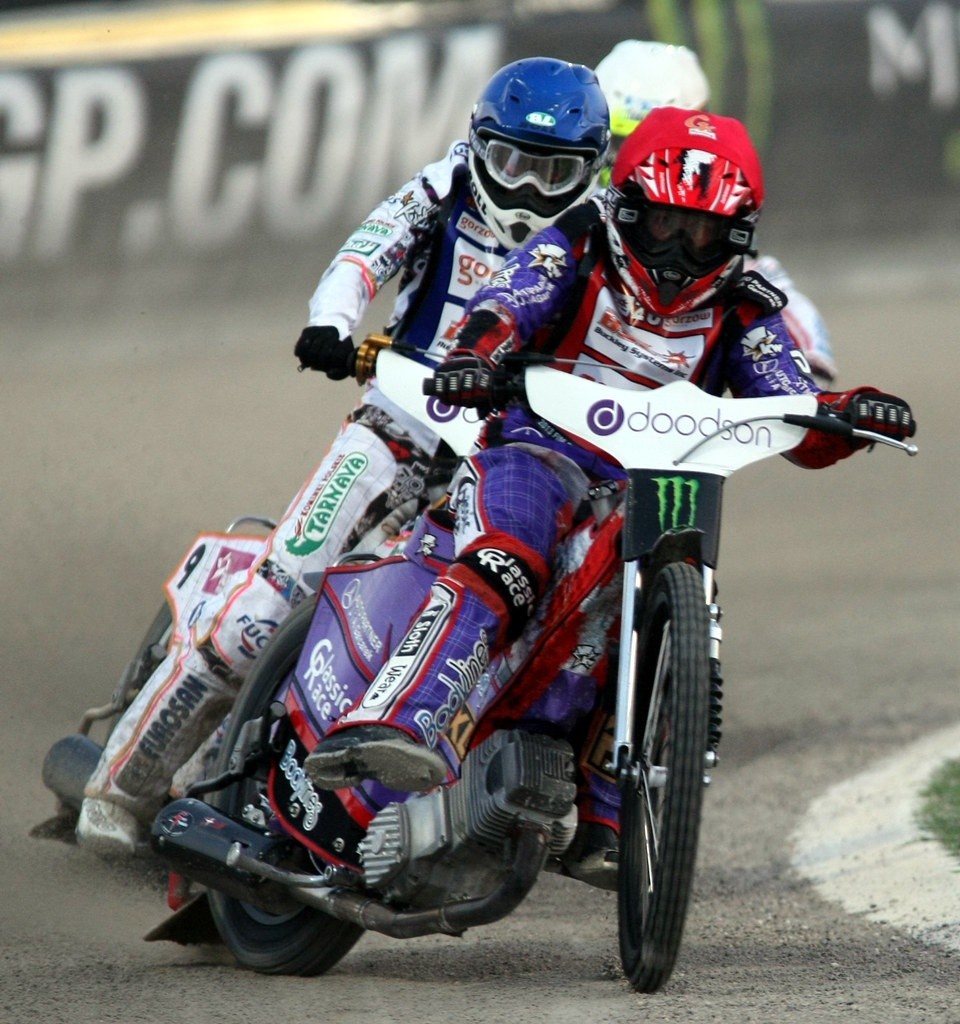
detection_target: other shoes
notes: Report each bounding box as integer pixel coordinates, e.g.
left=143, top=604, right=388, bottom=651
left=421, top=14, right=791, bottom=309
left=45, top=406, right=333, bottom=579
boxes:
left=571, top=825, right=622, bottom=881
left=303, top=729, right=445, bottom=794
left=75, top=796, right=139, bottom=864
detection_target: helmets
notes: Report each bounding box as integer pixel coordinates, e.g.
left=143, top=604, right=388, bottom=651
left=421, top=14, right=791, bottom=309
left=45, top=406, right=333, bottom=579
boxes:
left=474, top=57, right=611, bottom=249
left=610, top=106, right=767, bottom=320
left=593, top=40, right=709, bottom=189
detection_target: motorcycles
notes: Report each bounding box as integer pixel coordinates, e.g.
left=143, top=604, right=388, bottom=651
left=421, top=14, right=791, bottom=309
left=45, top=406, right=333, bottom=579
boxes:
left=141, top=344, right=921, bottom=994
left=25, top=333, right=487, bottom=891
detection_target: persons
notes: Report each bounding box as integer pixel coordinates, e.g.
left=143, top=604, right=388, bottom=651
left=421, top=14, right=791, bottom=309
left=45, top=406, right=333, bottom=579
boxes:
left=77, top=39, right=911, bottom=877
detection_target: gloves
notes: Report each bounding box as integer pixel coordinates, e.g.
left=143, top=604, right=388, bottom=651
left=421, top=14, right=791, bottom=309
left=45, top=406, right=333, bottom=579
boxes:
left=844, top=391, right=916, bottom=450
left=434, top=355, right=492, bottom=408
left=294, top=326, right=354, bottom=380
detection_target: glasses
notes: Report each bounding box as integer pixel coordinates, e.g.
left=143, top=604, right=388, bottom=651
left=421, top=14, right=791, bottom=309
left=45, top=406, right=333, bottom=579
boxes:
left=468, top=118, right=610, bottom=208
left=605, top=186, right=763, bottom=267
